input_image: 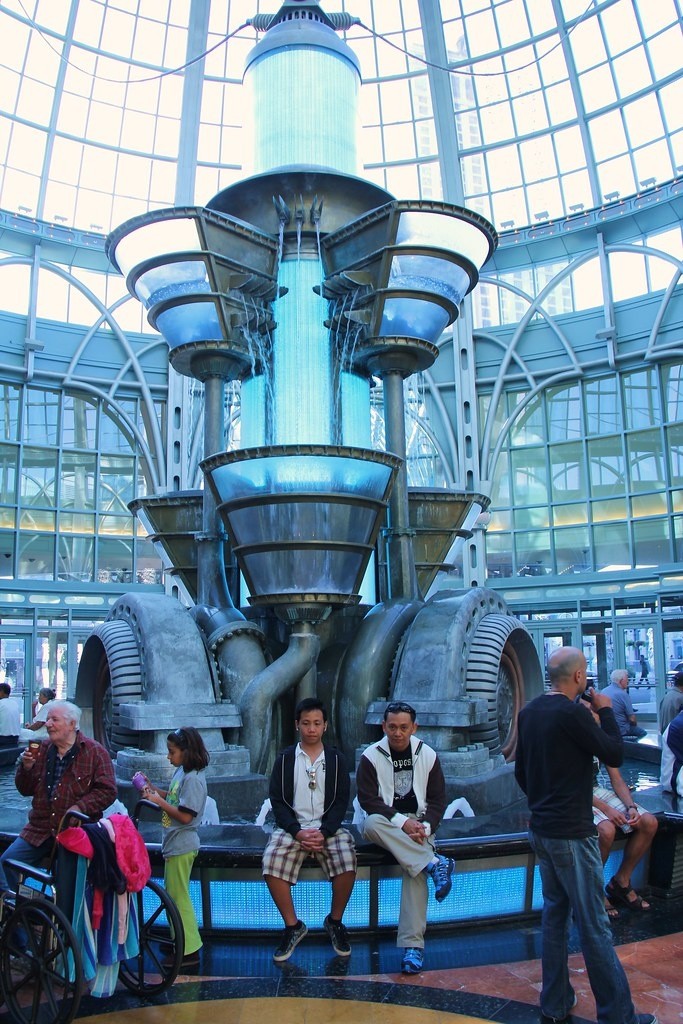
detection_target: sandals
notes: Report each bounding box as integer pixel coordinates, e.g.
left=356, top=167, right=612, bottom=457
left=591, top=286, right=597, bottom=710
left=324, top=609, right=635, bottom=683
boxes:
left=605, top=876, right=649, bottom=911
left=602, top=892, right=621, bottom=918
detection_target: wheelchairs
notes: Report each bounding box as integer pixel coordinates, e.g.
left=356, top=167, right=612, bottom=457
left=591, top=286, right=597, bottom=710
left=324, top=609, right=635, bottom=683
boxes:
left=0, top=797, right=185, bottom=1024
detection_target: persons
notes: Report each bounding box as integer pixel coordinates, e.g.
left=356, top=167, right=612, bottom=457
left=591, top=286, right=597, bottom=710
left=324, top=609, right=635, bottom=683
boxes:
left=263, top=698, right=356, bottom=962
left=356, top=702, right=455, bottom=974
left=535, top=561, right=547, bottom=575
left=602, top=669, right=648, bottom=743
left=0, top=683, right=20, bottom=748
left=659, top=705, right=683, bottom=821
left=576, top=696, right=658, bottom=920
left=634, top=655, right=651, bottom=689
left=515, top=647, right=658, bottom=1024
left=134, top=726, right=208, bottom=967
left=0, top=701, right=116, bottom=955
left=25, top=688, right=57, bottom=731
left=659, top=671, right=683, bottom=732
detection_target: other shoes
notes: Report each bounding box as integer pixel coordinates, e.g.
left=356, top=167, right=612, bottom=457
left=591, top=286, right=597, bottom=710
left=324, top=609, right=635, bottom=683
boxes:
left=159, top=950, right=201, bottom=968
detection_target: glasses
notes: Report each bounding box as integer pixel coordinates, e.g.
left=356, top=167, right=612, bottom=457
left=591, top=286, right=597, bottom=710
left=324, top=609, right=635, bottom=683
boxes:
left=387, top=703, right=413, bottom=715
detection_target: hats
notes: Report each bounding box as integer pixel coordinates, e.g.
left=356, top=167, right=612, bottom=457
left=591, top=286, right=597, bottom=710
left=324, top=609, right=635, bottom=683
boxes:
left=40, top=688, right=56, bottom=700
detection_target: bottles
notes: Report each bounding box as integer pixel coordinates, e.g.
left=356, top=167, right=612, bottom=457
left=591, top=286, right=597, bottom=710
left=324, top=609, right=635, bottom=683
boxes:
left=132, top=773, right=155, bottom=797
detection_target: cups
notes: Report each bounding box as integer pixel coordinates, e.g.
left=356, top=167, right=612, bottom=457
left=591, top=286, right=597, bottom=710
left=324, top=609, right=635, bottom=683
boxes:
left=28, top=738, right=42, bottom=758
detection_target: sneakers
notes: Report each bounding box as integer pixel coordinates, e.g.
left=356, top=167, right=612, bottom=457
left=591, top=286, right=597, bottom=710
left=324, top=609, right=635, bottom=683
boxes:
left=402, top=946, right=422, bottom=974
left=427, top=853, right=455, bottom=901
left=634, top=1013, right=657, bottom=1024
left=272, top=920, right=309, bottom=961
left=541, top=993, right=577, bottom=1018
left=325, top=957, right=349, bottom=983
left=398, top=974, right=422, bottom=1005
left=275, top=962, right=308, bottom=983
left=323, top=914, right=352, bottom=957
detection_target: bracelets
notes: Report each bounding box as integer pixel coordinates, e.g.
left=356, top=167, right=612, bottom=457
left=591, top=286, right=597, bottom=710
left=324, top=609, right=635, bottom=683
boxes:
left=627, top=806, right=637, bottom=812
